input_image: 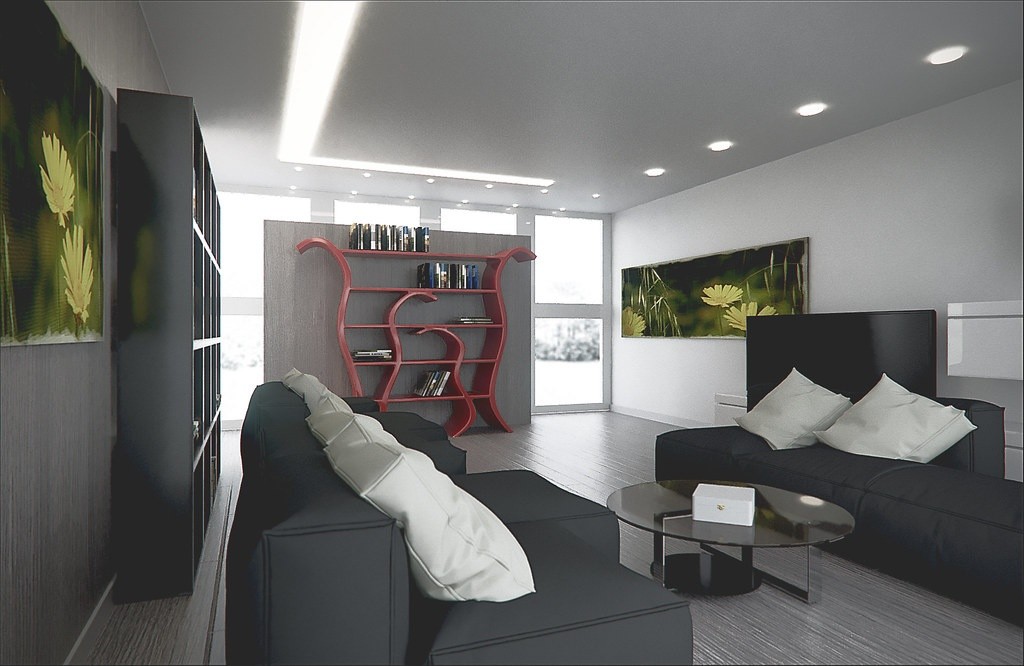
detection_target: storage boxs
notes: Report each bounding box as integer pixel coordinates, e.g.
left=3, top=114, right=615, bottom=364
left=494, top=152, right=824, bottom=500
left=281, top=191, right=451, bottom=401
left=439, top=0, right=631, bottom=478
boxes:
left=693, top=484, right=756, bottom=526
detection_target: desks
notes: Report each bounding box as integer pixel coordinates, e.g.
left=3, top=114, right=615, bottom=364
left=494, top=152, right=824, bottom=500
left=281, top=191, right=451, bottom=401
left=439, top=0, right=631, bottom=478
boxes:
left=607, top=478, right=864, bottom=602
left=714, top=390, right=746, bottom=427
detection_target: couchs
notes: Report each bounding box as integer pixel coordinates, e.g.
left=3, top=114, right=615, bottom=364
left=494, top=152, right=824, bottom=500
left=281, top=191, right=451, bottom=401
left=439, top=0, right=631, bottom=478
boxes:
left=654, top=397, right=1024, bottom=629
left=225, top=381, right=693, bottom=666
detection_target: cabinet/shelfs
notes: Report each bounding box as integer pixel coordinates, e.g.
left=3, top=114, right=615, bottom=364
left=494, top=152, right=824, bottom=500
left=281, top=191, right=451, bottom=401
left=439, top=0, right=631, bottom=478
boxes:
left=296, top=237, right=537, bottom=437
left=113, top=87, right=222, bottom=605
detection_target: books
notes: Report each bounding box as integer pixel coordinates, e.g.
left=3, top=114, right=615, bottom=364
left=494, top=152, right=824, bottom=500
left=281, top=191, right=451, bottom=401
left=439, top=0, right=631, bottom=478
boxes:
left=412, top=370, right=452, bottom=397
left=351, top=348, right=393, bottom=362
left=455, top=316, right=494, bottom=325
left=351, top=223, right=431, bottom=254
left=417, top=262, right=481, bottom=289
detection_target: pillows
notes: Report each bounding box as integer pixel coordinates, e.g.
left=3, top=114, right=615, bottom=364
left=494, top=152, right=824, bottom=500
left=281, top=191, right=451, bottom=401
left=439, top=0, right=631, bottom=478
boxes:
left=283, top=367, right=534, bottom=608
left=732, top=367, right=854, bottom=450
left=811, top=372, right=978, bottom=463
